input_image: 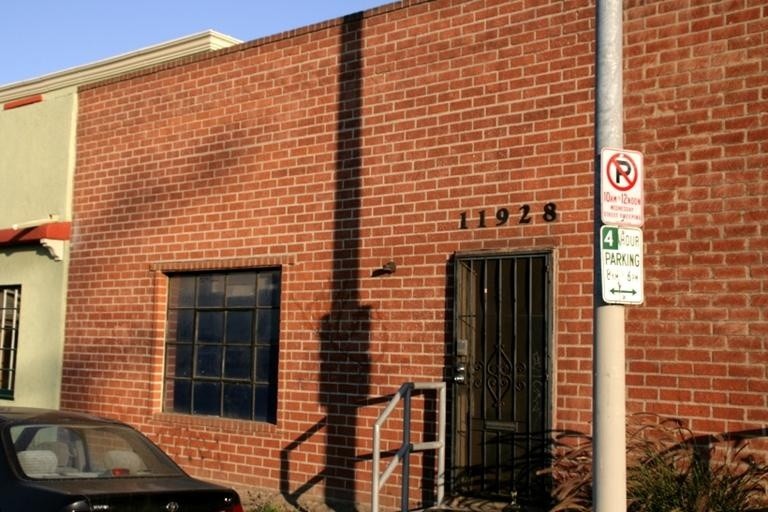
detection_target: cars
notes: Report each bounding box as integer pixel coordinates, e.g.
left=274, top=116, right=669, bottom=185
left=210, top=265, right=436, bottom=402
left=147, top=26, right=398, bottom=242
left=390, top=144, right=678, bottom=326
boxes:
left=0, top=406, right=244, bottom=512
